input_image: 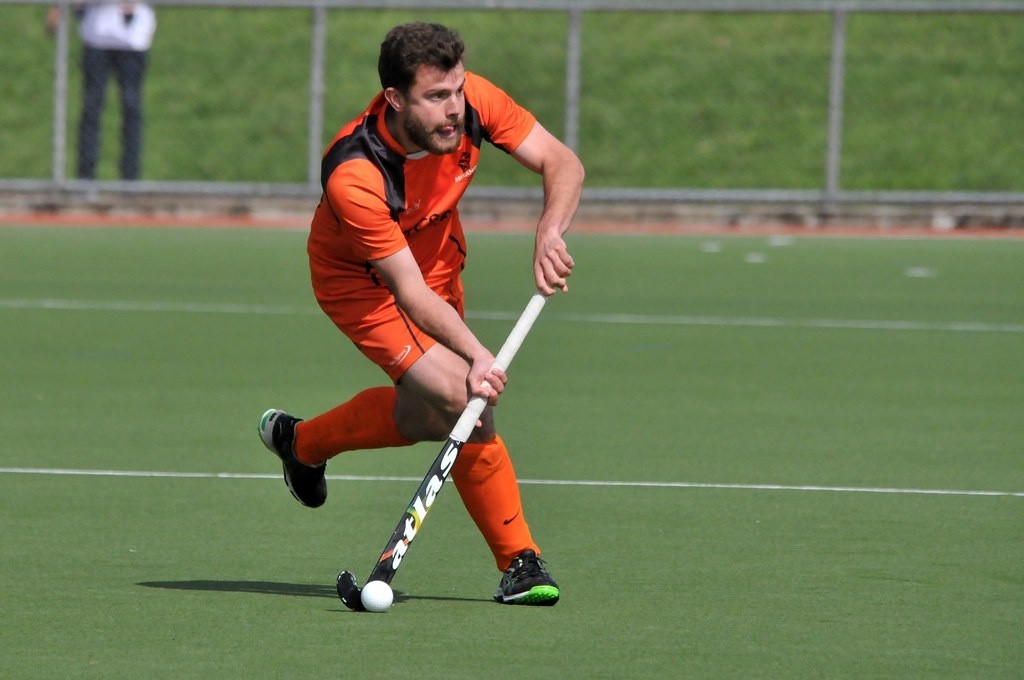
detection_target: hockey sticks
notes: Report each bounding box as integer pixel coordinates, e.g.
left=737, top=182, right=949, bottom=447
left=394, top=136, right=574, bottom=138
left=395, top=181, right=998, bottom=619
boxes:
left=335, top=252, right=572, bottom=613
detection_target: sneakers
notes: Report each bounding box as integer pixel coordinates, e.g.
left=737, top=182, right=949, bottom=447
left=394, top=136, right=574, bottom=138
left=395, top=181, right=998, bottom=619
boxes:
left=258, top=409, right=327, bottom=508
left=493, top=549, right=560, bottom=606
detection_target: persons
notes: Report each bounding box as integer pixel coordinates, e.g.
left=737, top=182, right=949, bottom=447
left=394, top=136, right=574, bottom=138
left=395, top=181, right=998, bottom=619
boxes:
left=45, top=0, right=157, bottom=182
left=259, top=23, right=585, bottom=607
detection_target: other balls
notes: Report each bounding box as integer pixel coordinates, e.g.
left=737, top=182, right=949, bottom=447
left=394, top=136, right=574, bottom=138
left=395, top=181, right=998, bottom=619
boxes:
left=360, top=580, right=395, bottom=612
